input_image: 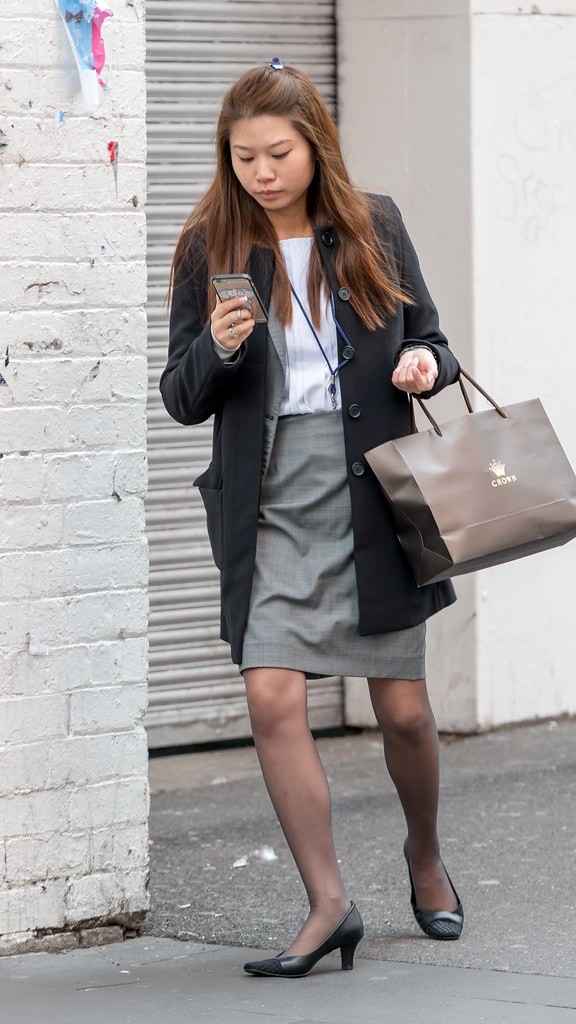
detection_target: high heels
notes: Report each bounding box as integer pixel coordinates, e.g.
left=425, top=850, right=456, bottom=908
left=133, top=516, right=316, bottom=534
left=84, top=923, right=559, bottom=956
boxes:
left=244, top=901, right=364, bottom=978
left=403, top=836, right=464, bottom=940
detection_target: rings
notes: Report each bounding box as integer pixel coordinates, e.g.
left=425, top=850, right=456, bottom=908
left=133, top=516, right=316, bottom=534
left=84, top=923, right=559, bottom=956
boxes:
left=229, top=329, right=241, bottom=339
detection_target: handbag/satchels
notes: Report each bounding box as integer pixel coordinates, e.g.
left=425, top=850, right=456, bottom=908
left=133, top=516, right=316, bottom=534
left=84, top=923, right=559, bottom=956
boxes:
left=363, top=342, right=576, bottom=588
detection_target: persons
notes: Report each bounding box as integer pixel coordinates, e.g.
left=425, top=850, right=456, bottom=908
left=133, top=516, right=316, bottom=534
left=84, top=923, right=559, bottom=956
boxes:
left=160, top=60, right=463, bottom=977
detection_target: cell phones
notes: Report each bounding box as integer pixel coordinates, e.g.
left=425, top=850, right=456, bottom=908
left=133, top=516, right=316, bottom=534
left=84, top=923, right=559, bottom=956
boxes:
left=211, top=274, right=270, bottom=323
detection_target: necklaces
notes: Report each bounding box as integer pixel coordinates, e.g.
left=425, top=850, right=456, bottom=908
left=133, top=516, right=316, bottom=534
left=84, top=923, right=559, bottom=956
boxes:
left=289, top=280, right=354, bottom=411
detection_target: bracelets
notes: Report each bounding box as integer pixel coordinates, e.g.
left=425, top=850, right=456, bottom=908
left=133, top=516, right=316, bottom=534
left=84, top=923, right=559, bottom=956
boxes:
left=398, top=344, right=440, bottom=367
